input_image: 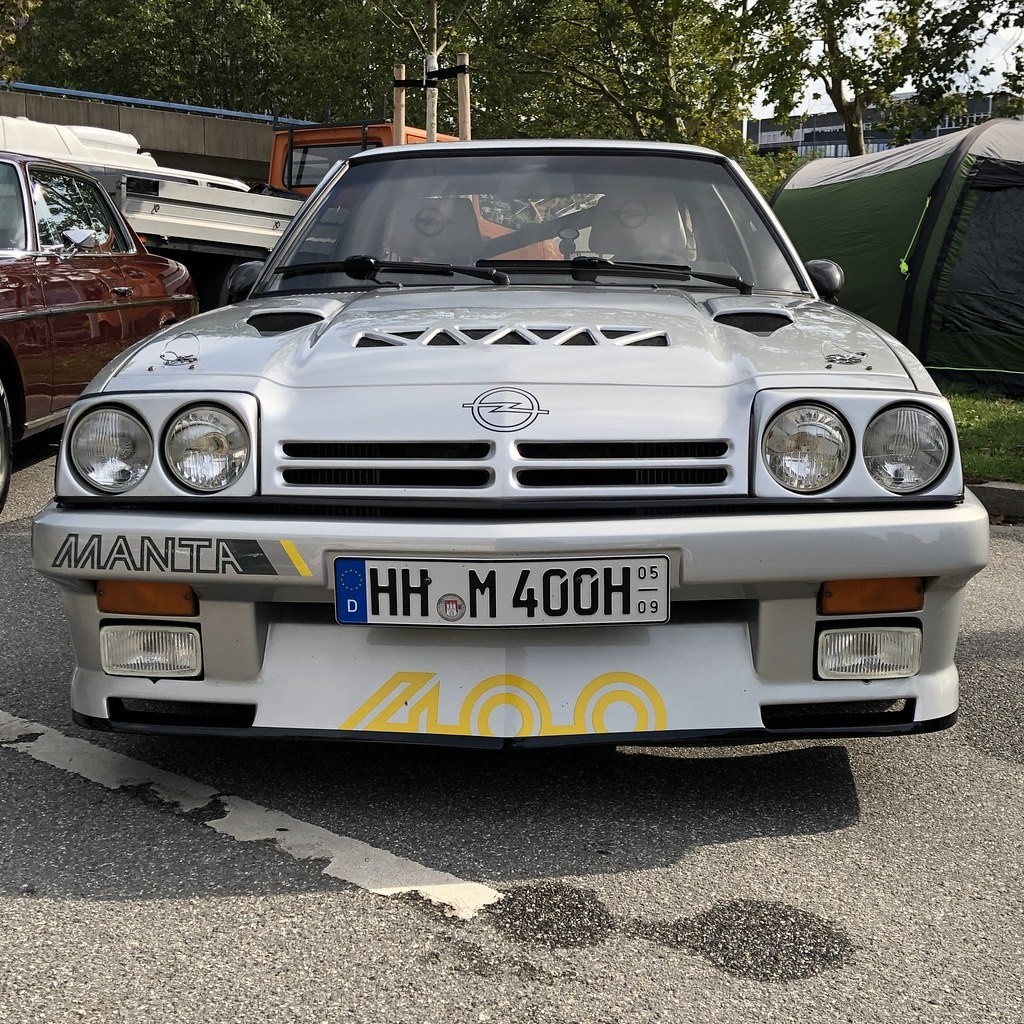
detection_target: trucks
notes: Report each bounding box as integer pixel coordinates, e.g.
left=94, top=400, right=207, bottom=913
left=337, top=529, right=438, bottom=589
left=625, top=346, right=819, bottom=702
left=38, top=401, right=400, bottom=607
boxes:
left=0, top=113, right=252, bottom=193
left=93, top=118, right=566, bottom=315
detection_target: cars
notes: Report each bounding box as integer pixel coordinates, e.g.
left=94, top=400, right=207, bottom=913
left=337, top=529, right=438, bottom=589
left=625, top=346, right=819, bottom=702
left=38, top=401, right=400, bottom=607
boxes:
left=0, top=148, right=200, bottom=513
left=28, top=137, right=992, bottom=750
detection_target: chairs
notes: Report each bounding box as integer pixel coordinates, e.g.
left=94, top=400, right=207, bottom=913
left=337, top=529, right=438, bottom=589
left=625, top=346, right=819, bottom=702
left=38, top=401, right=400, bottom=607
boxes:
left=387, top=196, right=483, bottom=260
left=588, top=191, right=686, bottom=258
